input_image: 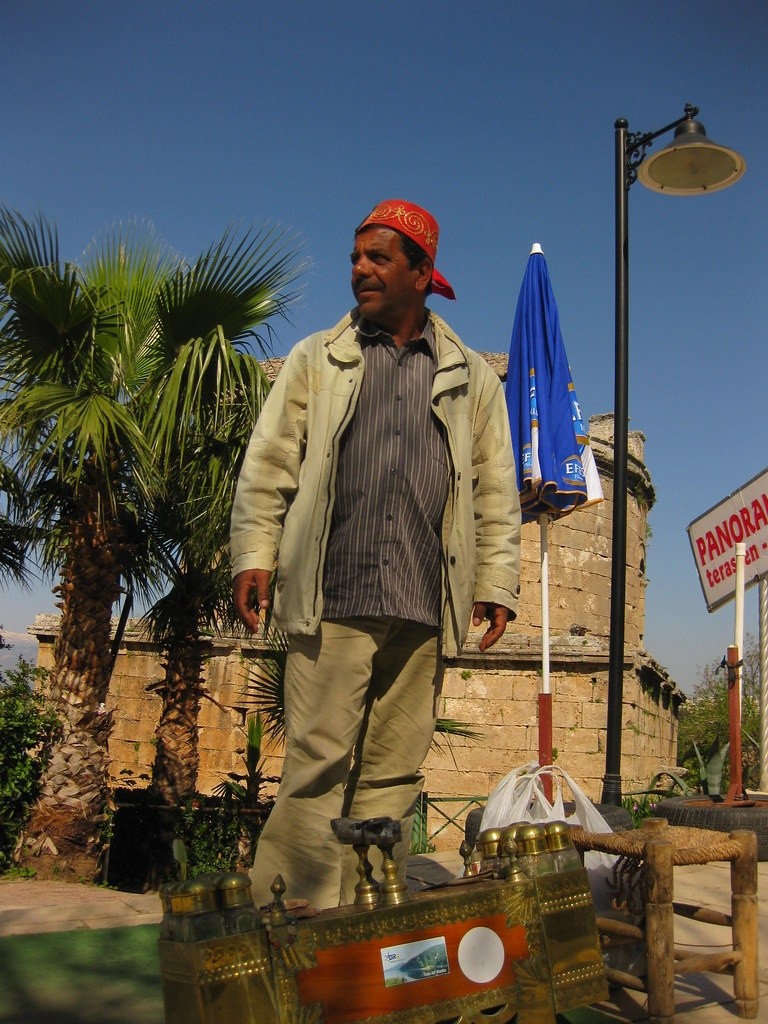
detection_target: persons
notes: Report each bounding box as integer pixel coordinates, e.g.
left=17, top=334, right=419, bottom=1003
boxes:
left=228, top=200, right=525, bottom=912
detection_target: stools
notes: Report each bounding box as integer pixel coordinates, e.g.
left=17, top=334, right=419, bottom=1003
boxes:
left=564, top=816, right=761, bottom=1018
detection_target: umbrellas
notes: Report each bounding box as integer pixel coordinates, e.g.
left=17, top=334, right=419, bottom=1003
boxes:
left=503, top=242, right=607, bottom=692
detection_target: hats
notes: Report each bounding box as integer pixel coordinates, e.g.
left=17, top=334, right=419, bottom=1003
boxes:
left=356, top=200, right=456, bottom=300
